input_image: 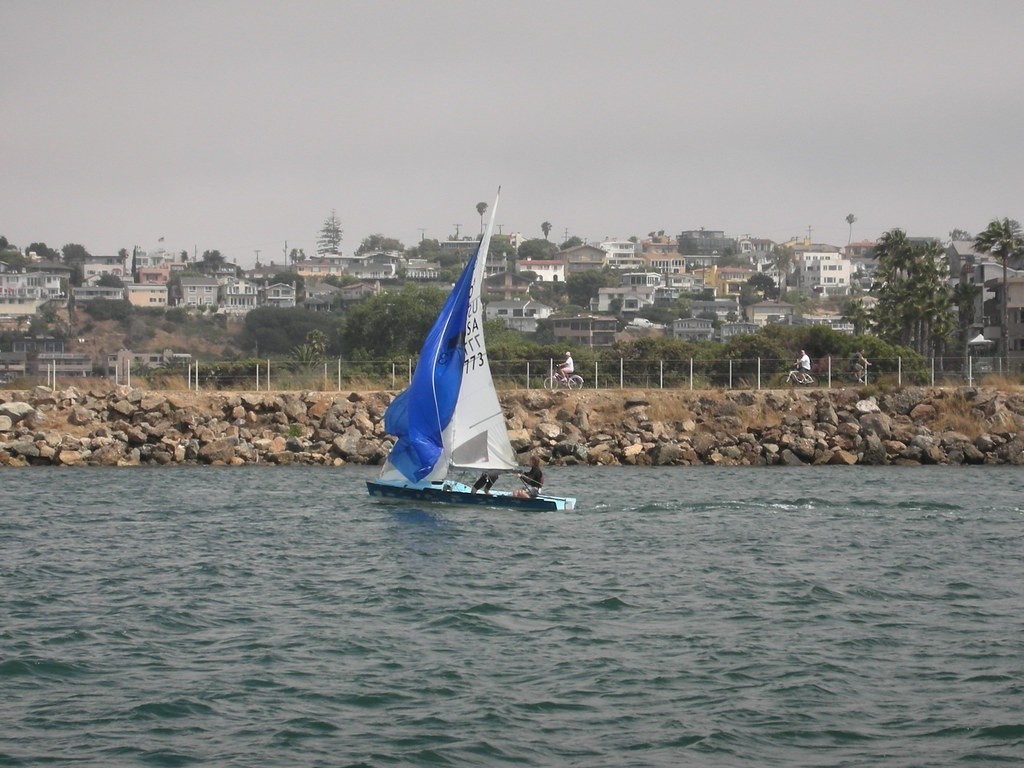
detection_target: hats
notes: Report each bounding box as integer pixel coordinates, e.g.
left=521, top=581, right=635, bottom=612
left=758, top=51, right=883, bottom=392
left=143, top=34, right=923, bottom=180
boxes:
left=566, top=352, right=570, bottom=356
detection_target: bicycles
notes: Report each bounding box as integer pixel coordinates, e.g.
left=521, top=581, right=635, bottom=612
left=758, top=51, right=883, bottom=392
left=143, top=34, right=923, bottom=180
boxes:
left=544, top=364, right=584, bottom=391
left=779, top=362, right=820, bottom=389
left=841, top=363, right=877, bottom=387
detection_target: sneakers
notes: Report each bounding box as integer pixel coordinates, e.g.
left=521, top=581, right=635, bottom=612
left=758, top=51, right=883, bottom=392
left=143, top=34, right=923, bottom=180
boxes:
left=560, top=378, right=567, bottom=381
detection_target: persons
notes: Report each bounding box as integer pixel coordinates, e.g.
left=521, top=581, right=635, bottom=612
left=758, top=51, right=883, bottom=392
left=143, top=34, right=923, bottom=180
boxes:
left=559, top=351, right=574, bottom=381
left=794, top=350, right=810, bottom=381
left=513, top=455, right=542, bottom=498
left=471, top=471, right=498, bottom=494
left=850, top=348, right=871, bottom=383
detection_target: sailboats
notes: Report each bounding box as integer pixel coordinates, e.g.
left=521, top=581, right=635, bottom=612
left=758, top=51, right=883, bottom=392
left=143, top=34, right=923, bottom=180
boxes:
left=365, top=181, right=578, bottom=510
